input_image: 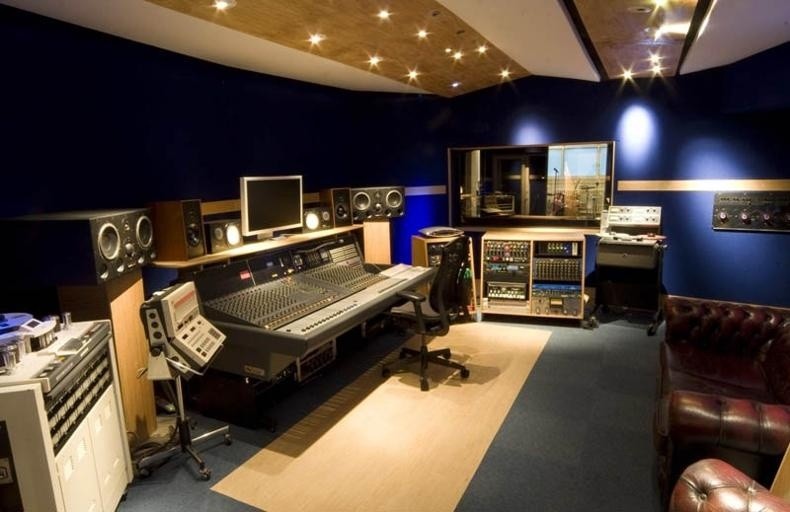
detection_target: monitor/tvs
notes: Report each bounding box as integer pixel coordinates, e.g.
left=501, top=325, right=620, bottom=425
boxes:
left=241, top=177, right=304, bottom=239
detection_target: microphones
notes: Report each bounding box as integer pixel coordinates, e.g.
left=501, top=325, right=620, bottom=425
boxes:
left=553, top=168, right=559, bottom=173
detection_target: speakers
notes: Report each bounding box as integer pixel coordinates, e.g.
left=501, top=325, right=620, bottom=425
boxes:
left=321, top=187, right=353, bottom=226
left=351, top=185, right=406, bottom=223
left=204, top=219, right=243, bottom=253
left=149, top=199, right=204, bottom=261
left=303, top=207, right=332, bottom=233
left=3, top=208, right=155, bottom=286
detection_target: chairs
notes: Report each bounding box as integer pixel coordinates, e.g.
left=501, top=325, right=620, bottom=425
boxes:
left=381, top=236, right=470, bottom=391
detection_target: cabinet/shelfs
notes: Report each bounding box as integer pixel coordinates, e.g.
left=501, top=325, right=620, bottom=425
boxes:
left=49, top=270, right=158, bottom=454
left=597, top=229, right=665, bottom=336
left=363, top=221, right=394, bottom=266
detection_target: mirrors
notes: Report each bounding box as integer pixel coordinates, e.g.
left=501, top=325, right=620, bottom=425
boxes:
left=449, top=139, right=616, bottom=231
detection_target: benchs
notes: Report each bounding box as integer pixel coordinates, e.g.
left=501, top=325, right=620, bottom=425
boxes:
left=653, top=295, right=790, bottom=512
